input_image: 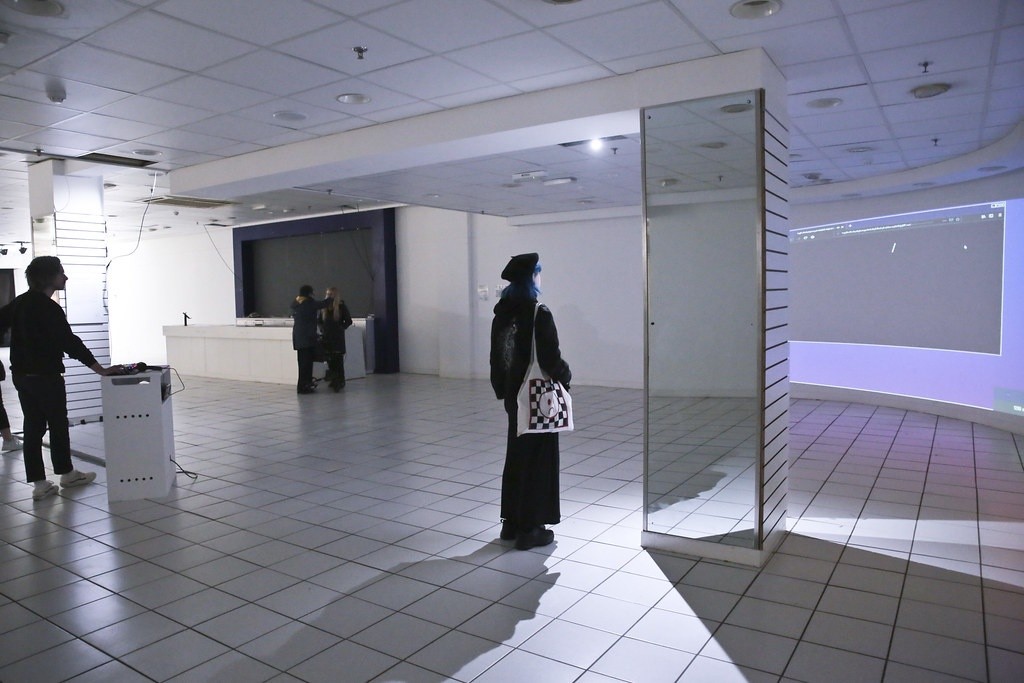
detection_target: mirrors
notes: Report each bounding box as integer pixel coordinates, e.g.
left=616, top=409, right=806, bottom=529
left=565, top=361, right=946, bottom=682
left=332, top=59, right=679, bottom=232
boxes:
left=642, top=87, right=767, bottom=551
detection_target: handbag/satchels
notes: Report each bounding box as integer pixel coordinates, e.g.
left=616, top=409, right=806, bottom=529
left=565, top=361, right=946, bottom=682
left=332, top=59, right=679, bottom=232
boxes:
left=516, top=303, right=574, bottom=437
left=311, top=339, right=332, bottom=362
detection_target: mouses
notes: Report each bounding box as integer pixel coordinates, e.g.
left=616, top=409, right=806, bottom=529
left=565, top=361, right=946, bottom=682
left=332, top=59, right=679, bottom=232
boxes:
left=109, top=368, right=129, bottom=376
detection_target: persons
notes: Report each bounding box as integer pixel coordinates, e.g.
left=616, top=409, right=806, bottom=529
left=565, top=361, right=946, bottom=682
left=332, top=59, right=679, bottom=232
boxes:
left=490, top=253, right=572, bottom=550
left=289, top=285, right=334, bottom=394
left=317, top=287, right=352, bottom=393
left=0, top=256, right=124, bottom=500
left=0, top=360, right=23, bottom=451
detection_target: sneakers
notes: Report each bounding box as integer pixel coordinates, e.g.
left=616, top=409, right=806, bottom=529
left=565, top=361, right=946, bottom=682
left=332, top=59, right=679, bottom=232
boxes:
left=32, top=480, right=59, bottom=502
left=499, top=518, right=546, bottom=541
left=60, top=469, right=97, bottom=488
left=1, top=436, right=24, bottom=451
left=514, top=525, right=555, bottom=550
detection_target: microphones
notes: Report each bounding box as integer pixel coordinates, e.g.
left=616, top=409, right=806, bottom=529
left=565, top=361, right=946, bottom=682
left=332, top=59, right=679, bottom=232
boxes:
left=137, top=362, right=164, bottom=372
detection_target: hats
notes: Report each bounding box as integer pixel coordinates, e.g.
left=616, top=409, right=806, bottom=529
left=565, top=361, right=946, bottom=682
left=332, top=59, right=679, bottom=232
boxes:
left=501, top=252, right=541, bottom=283
left=26, top=257, right=60, bottom=290
